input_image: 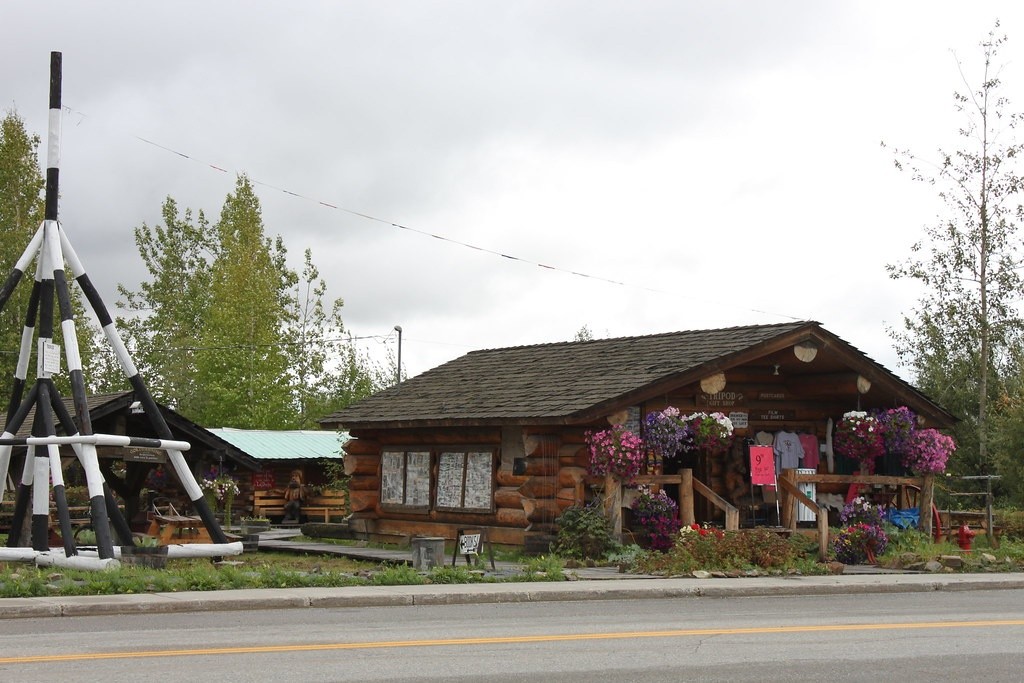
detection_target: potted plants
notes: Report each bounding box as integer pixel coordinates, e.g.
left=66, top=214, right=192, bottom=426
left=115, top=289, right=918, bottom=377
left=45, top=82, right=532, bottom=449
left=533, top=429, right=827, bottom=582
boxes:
left=240, top=516, right=271, bottom=535
left=110, top=460, right=126, bottom=479
left=549, top=503, right=620, bottom=559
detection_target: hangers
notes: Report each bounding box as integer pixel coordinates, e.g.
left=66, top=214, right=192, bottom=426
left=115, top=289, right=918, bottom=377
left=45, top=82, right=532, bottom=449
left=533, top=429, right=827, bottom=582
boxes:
left=802, top=425, right=812, bottom=435
left=781, top=424, right=793, bottom=434
left=744, top=432, right=752, bottom=439
left=762, top=423, right=771, bottom=433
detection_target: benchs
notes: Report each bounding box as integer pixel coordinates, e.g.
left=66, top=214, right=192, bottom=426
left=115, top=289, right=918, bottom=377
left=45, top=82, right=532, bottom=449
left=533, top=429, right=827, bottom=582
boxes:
left=935, top=524, right=1002, bottom=535
left=223, top=531, right=243, bottom=541
left=134, top=532, right=156, bottom=541
left=254, top=490, right=346, bottom=521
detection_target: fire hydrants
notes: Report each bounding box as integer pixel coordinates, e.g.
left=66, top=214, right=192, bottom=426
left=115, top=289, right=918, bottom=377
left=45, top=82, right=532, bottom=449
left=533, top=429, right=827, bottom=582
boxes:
left=956, top=524, right=976, bottom=555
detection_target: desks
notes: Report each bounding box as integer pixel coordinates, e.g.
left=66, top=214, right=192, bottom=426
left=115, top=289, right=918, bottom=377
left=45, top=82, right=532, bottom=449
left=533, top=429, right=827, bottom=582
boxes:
left=147, top=516, right=215, bottom=546
left=937, top=509, right=998, bottom=549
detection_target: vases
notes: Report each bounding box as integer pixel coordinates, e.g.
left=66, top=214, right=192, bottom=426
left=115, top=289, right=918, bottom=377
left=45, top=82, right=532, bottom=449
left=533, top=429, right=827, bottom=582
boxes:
left=649, top=534, right=673, bottom=548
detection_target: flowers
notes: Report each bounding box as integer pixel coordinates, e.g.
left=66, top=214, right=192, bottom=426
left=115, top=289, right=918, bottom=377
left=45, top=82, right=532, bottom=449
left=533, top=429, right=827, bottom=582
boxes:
left=583, top=407, right=735, bottom=481
left=833, top=406, right=955, bottom=477
left=145, top=465, right=167, bottom=488
left=197, top=474, right=240, bottom=501
left=632, top=484, right=682, bottom=544
left=832, top=496, right=890, bottom=566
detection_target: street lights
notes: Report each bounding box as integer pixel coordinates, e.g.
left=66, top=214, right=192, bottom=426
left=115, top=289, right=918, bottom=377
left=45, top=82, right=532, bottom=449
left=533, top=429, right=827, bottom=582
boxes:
left=395, top=324, right=403, bottom=384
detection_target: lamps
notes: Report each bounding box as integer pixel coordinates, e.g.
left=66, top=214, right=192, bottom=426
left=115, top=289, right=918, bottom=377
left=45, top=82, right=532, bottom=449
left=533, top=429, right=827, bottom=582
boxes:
left=773, top=364, right=780, bottom=376
